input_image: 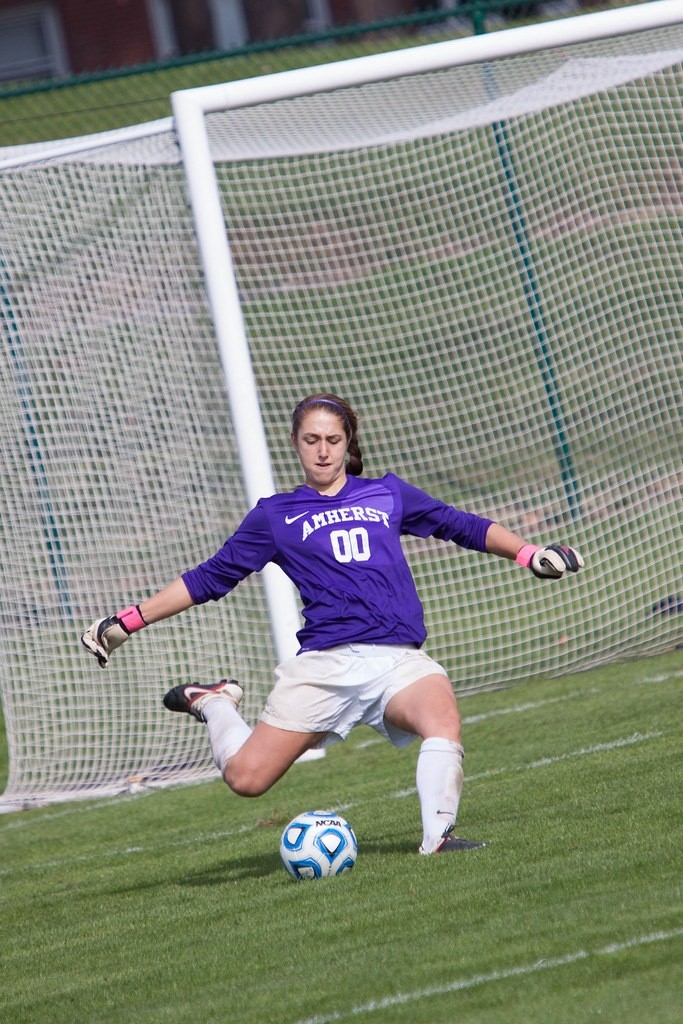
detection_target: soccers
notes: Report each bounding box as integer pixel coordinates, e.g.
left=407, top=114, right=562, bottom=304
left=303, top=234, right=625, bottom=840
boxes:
left=279, top=811, right=359, bottom=882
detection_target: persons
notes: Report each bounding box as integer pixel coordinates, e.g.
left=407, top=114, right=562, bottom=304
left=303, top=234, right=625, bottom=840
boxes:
left=83, top=391, right=586, bottom=854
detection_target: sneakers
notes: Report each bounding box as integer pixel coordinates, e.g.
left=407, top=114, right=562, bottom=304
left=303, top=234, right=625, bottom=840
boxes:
left=163, top=678, right=244, bottom=724
left=419, top=823, right=496, bottom=856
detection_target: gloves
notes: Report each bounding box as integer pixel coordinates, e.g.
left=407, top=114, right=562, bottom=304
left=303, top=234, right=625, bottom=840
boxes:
left=80, top=604, right=149, bottom=669
left=515, top=543, right=585, bottom=579
